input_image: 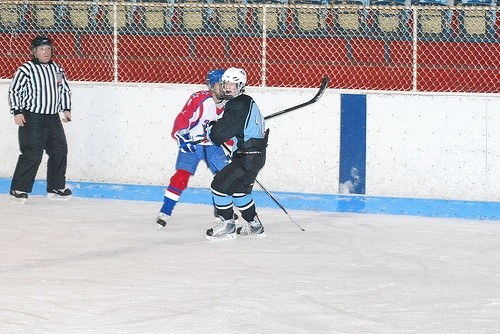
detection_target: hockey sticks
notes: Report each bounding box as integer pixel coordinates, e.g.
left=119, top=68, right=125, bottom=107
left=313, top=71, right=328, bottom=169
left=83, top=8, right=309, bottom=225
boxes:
left=222, top=143, right=306, bottom=231
left=192, top=76, right=329, bottom=145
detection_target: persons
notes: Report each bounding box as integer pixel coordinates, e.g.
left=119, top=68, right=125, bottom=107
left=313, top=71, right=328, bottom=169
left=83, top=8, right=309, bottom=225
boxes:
left=8, top=38, right=73, bottom=203
left=206, top=67, right=269, bottom=242
left=156, top=70, right=234, bottom=231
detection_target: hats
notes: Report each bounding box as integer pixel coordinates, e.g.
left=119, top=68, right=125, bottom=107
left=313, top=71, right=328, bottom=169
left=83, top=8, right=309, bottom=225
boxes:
left=31, top=36, right=53, bottom=47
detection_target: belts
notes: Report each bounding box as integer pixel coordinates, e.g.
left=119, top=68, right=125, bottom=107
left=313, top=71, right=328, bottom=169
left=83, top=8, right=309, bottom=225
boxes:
left=237, top=149, right=266, bottom=155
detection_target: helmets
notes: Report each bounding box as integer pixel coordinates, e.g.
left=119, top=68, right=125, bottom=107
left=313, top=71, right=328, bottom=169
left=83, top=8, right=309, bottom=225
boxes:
left=206, top=69, right=225, bottom=92
left=221, top=67, right=247, bottom=95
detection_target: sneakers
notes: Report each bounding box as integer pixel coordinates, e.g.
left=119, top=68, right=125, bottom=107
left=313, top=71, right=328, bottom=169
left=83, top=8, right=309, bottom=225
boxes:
left=156, top=212, right=170, bottom=229
left=47, top=187, right=72, bottom=200
left=8, top=190, right=28, bottom=203
left=237, top=216, right=268, bottom=238
left=214, top=207, right=239, bottom=224
left=205, top=216, right=237, bottom=241
